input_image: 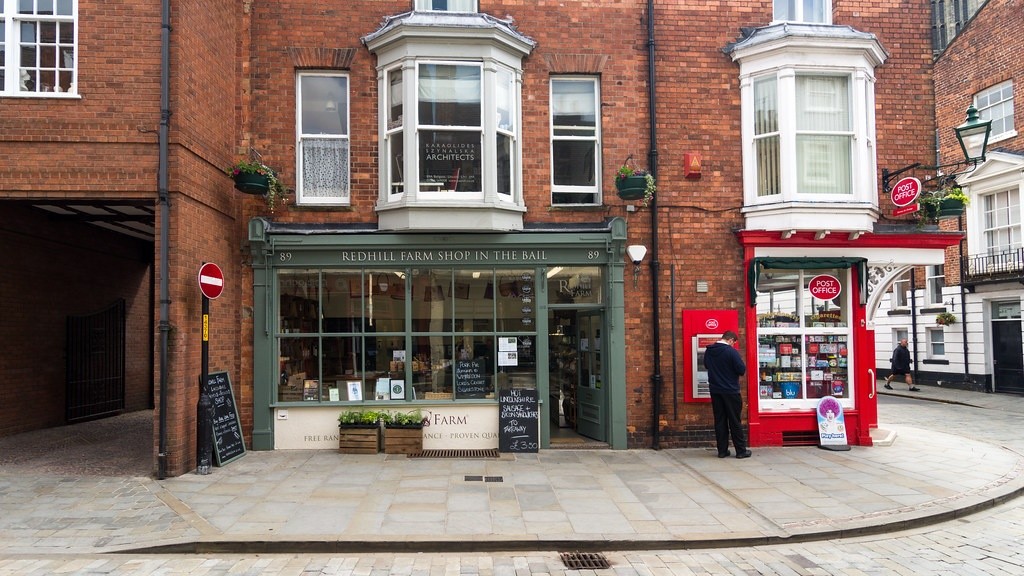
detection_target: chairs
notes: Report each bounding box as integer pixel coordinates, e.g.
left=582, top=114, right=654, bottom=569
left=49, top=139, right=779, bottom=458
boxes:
left=442, top=167, right=460, bottom=192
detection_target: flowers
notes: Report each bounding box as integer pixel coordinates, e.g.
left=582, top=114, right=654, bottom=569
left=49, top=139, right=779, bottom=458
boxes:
left=226, top=156, right=286, bottom=215
left=611, top=164, right=656, bottom=207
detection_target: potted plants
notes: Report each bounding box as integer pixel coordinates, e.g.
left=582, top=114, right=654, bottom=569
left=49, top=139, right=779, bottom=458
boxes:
left=915, top=186, right=969, bottom=229
left=936, top=312, right=957, bottom=327
left=337, top=408, right=425, bottom=454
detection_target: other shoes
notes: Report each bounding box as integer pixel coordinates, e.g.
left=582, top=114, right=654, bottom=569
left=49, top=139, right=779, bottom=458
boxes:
left=909, top=387, right=920, bottom=391
left=884, top=384, right=892, bottom=389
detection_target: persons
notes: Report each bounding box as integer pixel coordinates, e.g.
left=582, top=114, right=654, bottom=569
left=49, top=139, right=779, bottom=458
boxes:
left=704, top=330, right=752, bottom=459
left=884, top=338, right=920, bottom=391
left=818, top=398, right=847, bottom=445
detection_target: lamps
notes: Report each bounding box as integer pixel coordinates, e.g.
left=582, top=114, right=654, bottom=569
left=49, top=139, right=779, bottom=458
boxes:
left=916, top=102, right=993, bottom=176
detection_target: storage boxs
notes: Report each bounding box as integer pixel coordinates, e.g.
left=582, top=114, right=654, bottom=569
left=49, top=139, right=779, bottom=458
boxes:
left=278, top=371, right=307, bottom=402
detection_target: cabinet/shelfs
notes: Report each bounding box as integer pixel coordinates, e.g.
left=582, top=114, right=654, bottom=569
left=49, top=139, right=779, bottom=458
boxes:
left=758, top=340, right=848, bottom=382
left=280, top=293, right=325, bottom=378
left=550, top=332, right=577, bottom=430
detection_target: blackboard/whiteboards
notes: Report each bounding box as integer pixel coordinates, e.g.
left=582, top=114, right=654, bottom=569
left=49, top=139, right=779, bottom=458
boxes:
left=455, top=359, right=486, bottom=399
left=199, top=370, right=247, bottom=466
left=499, top=390, right=539, bottom=453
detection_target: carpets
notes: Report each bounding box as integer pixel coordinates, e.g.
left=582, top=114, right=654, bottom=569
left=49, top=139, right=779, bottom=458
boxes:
left=551, top=437, right=585, bottom=443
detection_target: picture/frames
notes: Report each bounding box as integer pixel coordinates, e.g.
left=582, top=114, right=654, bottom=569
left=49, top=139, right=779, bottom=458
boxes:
left=303, top=379, right=336, bottom=402
left=388, top=377, right=406, bottom=400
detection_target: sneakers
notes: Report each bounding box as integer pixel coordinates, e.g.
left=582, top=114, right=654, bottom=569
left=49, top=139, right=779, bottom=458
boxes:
left=736, top=450, right=751, bottom=458
left=718, top=450, right=730, bottom=458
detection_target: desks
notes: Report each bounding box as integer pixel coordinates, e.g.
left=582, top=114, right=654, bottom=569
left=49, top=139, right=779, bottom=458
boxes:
left=318, top=372, right=385, bottom=394
left=387, top=365, right=451, bottom=388
left=392, top=182, right=445, bottom=192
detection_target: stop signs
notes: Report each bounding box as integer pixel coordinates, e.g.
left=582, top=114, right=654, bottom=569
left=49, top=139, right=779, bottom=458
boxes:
left=197, top=262, right=224, bottom=300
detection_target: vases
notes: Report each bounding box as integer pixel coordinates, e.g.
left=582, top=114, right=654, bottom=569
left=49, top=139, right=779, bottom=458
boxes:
left=617, top=176, right=647, bottom=200
left=232, top=173, right=269, bottom=194
